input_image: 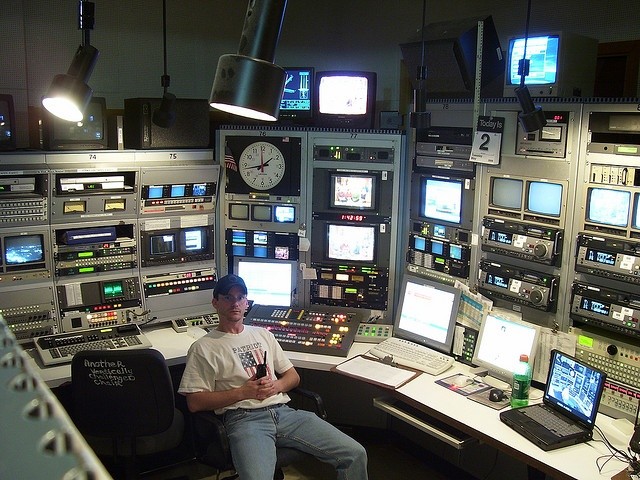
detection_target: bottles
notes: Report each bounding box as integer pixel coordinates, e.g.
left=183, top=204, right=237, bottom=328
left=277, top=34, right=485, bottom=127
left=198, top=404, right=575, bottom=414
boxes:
left=511, top=355, right=532, bottom=407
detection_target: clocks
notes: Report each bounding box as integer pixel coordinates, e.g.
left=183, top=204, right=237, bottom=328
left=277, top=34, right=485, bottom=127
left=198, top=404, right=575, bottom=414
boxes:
left=238, top=142, right=286, bottom=192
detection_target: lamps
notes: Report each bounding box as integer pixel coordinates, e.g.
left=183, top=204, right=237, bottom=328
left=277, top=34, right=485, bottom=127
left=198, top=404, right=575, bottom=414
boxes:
left=512, top=0, right=548, bottom=133
left=152, top=2, right=178, bottom=128
left=207, top=0, right=288, bottom=121
left=40, top=0, right=100, bottom=123
left=408, top=0, right=431, bottom=129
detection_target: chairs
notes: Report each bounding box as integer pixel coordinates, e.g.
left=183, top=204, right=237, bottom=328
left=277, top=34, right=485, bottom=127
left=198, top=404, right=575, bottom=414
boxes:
left=71, top=349, right=185, bottom=480
left=191, top=387, right=327, bottom=480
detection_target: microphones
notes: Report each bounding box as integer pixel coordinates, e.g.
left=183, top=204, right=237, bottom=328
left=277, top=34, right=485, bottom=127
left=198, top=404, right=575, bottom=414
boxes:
left=126, top=305, right=150, bottom=317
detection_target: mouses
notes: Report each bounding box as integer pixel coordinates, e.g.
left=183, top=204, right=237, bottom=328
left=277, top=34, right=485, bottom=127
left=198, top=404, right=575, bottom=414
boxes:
left=453, top=375, right=475, bottom=388
left=489, top=388, right=503, bottom=401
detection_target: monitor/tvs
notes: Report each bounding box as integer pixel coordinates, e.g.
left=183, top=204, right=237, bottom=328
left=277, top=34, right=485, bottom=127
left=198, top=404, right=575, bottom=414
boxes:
left=277, top=67, right=314, bottom=118
left=588, top=189, right=630, bottom=228
left=323, top=221, right=378, bottom=266
left=471, top=311, right=542, bottom=380
left=233, top=257, right=297, bottom=307
left=142, top=230, right=180, bottom=265
left=0, top=94, right=16, bottom=151
left=330, top=174, right=376, bottom=210
left=632, top=193, right=640, bottom=230
left=503, top=31, right=598, bottom=98
left=181, top=228, right=209, bottom=256
left=394, top=274, right=462, bottom=354
left=492, top=178, right=523, bottom=210
left=1, top=231, right=50, bottom=274
left=527, top=181, right=562, bottom=217
left=315, top=70, right=377, bottom=129
left=42, top=97, right=108, bottom=150
left=418, top=176, right=463, bottom=224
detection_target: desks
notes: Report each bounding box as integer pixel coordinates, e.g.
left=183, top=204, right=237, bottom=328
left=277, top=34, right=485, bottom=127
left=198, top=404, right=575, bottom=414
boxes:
left=24, top=293, right=632, bottom=480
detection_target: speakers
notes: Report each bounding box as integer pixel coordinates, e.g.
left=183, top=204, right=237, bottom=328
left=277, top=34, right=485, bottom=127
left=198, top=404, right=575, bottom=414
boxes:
left=399, top=14, right=505, bottom=96
left=124, top=99, right=212, bottom=149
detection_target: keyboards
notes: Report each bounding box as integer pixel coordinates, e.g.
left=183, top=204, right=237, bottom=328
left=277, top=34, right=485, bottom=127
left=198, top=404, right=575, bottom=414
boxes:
left=370, top=336, right=455, bottom=375
left=33, top=323, right=152, bottom=366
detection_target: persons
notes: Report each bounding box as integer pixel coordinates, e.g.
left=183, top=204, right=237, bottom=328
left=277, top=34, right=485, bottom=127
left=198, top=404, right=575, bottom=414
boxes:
left=177, top=275, right=369, bottom=480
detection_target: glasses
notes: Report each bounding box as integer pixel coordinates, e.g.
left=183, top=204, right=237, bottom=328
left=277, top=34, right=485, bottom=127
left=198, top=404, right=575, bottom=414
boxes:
left=218, top=293, right=247, bottom=304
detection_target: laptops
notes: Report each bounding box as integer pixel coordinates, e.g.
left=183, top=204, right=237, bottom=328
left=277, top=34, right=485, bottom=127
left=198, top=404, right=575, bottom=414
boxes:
left=500, top=348, right=608, bottom=452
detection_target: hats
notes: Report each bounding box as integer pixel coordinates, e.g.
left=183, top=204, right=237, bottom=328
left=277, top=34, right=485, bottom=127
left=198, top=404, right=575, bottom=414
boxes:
left=213, top=274, right=247, bottom=298
